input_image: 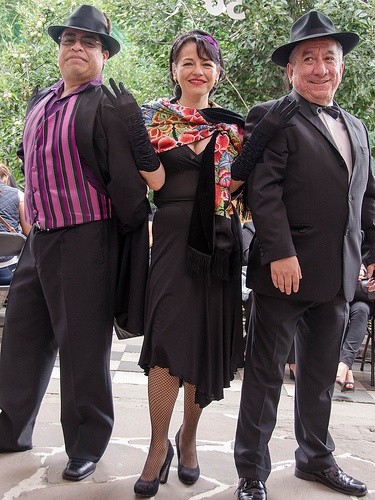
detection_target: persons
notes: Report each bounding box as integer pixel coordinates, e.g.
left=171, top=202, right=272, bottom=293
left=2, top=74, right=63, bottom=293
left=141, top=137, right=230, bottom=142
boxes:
left=235, top=10, right=375, bottom=500
left=0, top=162, right=33, bottom=285
left=0, top=4, right=151, bottom=481
left=240, top=221, right=375, bottom=392
left=99, top=29, right=301, bottom=497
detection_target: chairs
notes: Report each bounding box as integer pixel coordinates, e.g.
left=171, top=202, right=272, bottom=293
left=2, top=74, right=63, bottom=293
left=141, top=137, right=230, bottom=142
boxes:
left=356, top=301, right=375, bottom=386
left=0, top=232, right=27, bottom=309
